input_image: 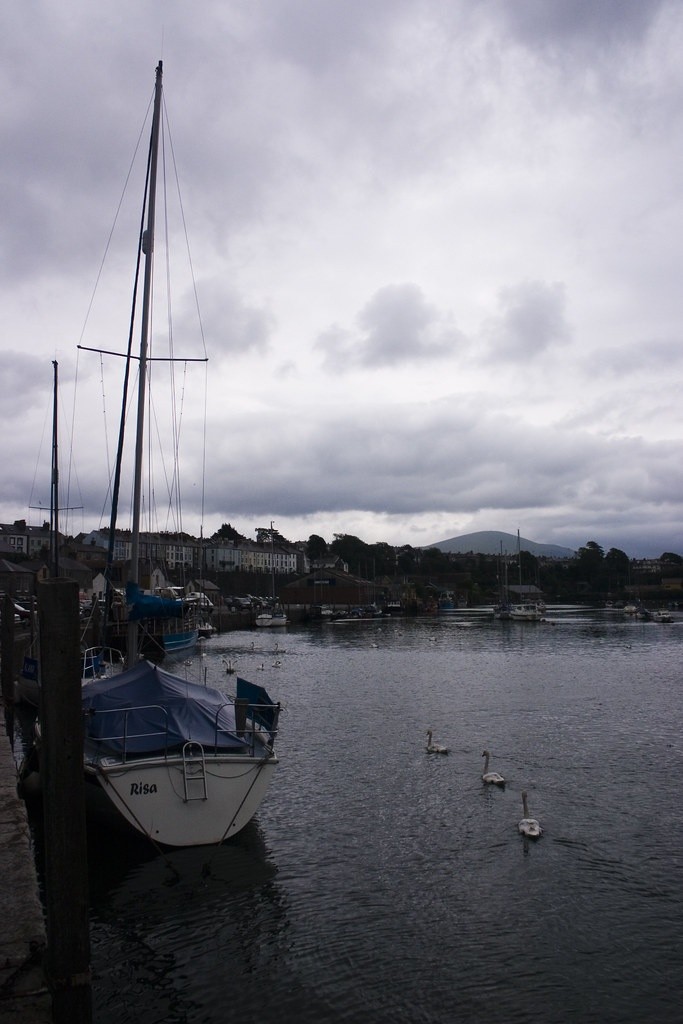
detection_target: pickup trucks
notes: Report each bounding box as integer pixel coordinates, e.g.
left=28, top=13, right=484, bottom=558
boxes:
left=226, top=598, right=252, bottom=612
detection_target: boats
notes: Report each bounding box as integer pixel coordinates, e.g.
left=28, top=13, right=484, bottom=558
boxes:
left=272, top=615, right=287, bottom=626
left=197, top=623, right=212, bottom=640
left=255, top=613, right=273, bottom=627
left=117, top=597, right=199, bottom=656
left=17, top=638, right=145, bottom=708
left=624, top=606, right=637, bottom=613
left=510, top=603, right=543, bottom=621
left=654, top=610, right=673, bottom=622
left=606, top=601, right=613, bottom=607
left=636, top=608, right=651, bottom=619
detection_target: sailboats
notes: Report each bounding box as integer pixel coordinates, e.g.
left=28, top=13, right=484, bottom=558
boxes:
left=80, top=58, right=282, bottom=846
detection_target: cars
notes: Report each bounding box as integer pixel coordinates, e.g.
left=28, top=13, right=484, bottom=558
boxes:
left=1, top=594, right=37, bottom=628
left=83, top=600, right=105, bottom=616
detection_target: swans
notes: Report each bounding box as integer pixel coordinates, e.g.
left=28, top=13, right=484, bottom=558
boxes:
left=482, top=749, right=505, bottom=785
left=518, top=790, right=545, bottom=840
left=426, top=730, right=448, bottom=753
